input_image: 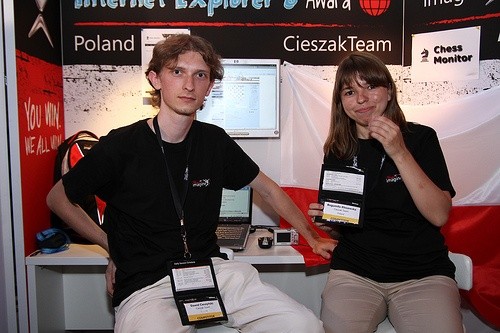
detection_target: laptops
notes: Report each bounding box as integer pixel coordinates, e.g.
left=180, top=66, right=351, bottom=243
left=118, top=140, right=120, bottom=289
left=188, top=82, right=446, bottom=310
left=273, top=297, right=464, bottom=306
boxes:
left=214, top=185, right=253, bottom=251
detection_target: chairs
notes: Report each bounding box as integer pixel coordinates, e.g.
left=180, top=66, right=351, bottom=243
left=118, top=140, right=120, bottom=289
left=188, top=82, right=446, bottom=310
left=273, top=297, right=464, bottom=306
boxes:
left=197, top=247, right=473, bottom=333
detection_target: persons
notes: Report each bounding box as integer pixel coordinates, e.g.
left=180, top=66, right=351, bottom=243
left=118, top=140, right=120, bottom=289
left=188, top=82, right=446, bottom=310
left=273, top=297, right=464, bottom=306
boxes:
left=308, top=50, right=462, bottom=333
left=47, top=34, right=339, bottom=333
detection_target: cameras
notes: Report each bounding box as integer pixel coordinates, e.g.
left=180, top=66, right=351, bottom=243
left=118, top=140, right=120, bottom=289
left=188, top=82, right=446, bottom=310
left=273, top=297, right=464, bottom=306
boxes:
left=273, top=228, right=299, bottom=246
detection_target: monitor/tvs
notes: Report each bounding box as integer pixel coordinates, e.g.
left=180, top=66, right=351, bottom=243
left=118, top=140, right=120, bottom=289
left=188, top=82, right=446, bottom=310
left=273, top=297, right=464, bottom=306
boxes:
left=193, top=59, right=280, bottom=138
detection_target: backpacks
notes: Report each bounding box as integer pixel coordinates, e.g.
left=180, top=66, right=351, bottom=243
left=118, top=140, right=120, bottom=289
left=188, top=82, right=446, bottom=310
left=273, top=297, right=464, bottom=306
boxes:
left=50, top=130, right=107, bottom=245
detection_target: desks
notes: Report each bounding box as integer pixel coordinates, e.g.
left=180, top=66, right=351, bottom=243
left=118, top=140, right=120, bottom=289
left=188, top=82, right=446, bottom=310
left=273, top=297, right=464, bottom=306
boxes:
left=25, top=228, right=330, bottom=333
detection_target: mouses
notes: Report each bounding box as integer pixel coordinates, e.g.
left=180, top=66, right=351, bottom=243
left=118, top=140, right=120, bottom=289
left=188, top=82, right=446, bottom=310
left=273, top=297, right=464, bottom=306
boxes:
left=258, top=237, right=272, bottom=248
